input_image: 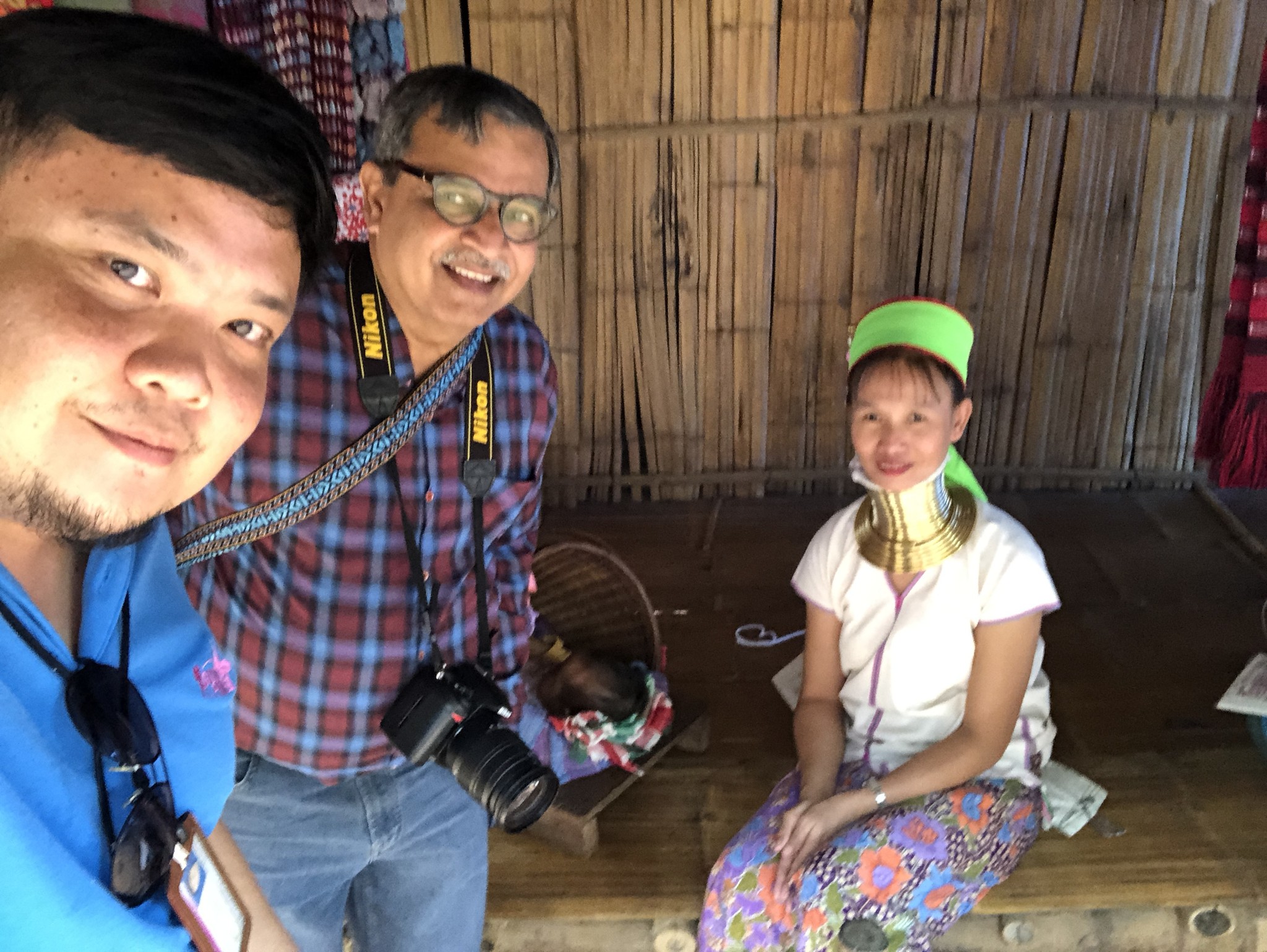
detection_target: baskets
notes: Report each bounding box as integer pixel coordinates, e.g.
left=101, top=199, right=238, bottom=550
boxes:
left=511, top=541, right=669, bottom=752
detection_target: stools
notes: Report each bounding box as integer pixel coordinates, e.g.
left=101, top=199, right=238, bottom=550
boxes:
left=525, top=702, right=716, bottom=857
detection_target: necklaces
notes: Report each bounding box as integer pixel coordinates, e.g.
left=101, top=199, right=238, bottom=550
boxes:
left=852, top=466, right=978, bottom=576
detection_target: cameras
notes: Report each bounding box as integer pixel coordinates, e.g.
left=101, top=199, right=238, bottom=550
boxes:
left=377, top=661, right=562, bottom=834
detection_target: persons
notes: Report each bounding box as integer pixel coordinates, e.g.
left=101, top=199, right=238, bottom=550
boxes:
left=2, top=5, right=338, bottom=952
left=701, top=330, right=1052, bottom=952
left=162, top=66, right=558, bottom=952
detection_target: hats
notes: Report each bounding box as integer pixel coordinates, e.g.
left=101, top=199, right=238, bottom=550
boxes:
left=848, top=296, right=977, bottom=384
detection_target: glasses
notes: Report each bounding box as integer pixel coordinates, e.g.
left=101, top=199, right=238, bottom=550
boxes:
left=65, top=657, right=179, bottom=909
left=378, top=155, right=560, bottom=243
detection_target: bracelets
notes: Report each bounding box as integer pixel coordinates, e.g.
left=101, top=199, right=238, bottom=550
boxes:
left=869, top=779, right=891, bottom=813
